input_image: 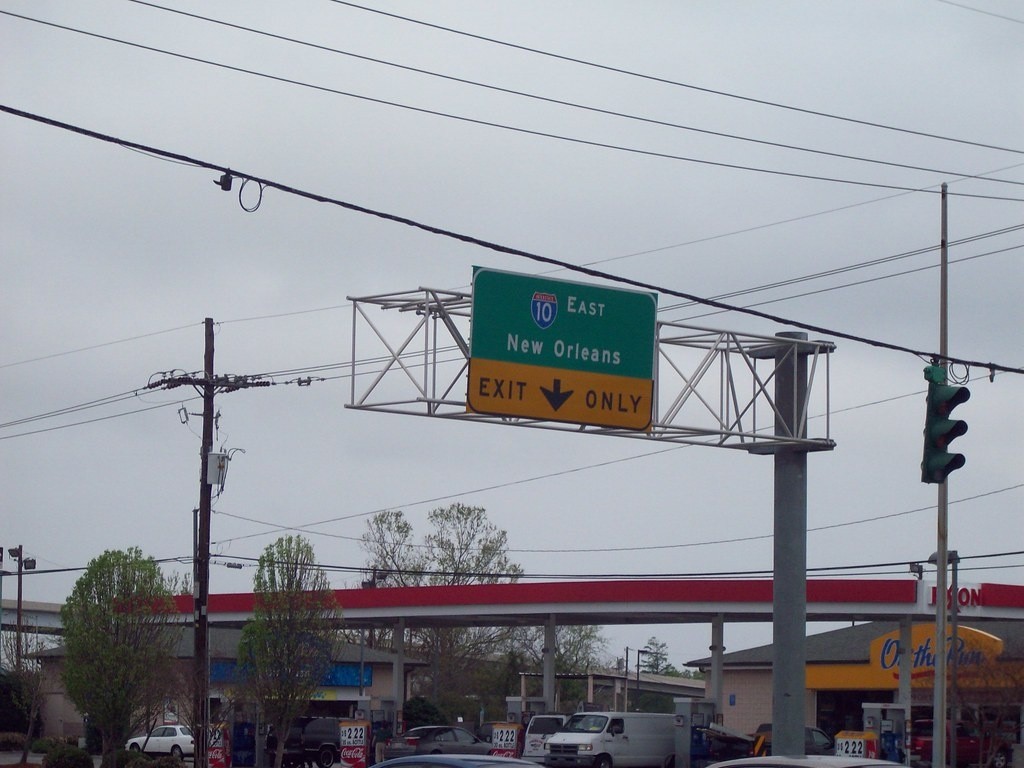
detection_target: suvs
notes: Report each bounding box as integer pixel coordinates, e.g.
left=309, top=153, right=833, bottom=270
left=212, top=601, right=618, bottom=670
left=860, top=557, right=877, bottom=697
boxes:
left=476, top=721, right=507, bottom=742
left=264, top=716, right=356, bottom=768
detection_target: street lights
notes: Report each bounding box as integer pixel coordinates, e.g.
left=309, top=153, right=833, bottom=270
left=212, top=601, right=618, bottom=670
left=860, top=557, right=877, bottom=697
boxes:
left=928, top=549, right=961, bottom=768
left=7, top=543, right=36, bottom=668
left=636, top=650, right=648, bottom=711
left=362, top=566, right=388, bottom=649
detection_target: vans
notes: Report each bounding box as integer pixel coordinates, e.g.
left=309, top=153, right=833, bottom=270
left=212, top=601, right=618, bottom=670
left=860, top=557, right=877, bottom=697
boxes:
left=543, top=711, right=677, bottom=768
left=520, top=715, right=573, bottom=762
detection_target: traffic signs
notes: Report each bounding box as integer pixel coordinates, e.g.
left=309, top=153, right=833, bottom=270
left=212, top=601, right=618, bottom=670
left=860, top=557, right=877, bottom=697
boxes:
left=468, top=264, right=656, bottom=432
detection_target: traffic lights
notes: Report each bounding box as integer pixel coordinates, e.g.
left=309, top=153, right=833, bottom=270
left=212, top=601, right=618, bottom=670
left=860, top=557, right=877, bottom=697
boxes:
left=923, top=385, right=971, bottom=484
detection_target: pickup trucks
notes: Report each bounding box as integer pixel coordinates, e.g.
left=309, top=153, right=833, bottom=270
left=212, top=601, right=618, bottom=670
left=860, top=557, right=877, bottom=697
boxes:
left=912, top=719, right=1014, bottom=768
left=706, top=722, right=834, bottom=765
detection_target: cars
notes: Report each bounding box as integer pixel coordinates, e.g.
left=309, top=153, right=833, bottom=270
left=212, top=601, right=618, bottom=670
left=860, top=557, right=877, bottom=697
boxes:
left=702, top=723, right=755, bottom=762
left=368, top=753, right=547, bottom=768
left=382, top=726, right=493, bottom=759
left=125, top=724, right=195, bottom=761
left=704, top=755, right=912, bottom=768
